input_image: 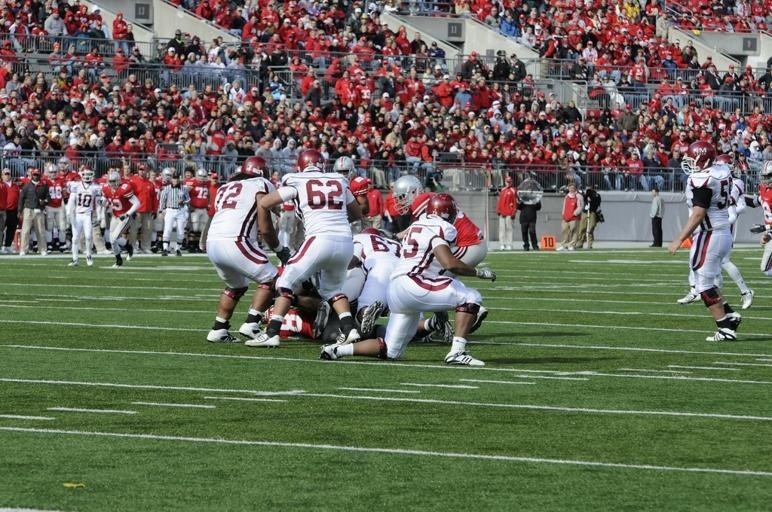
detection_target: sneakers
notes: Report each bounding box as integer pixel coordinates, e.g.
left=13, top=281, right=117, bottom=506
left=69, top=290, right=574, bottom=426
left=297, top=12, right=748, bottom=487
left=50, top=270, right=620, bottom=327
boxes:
left=678, top=288, right=701, bottom=303
left=207, top=301, right=488, bottom=366
left=500, top=242, right=590, bottom=251
left=741, top=290, right=754, bottom=309
left=706, top=311, right=741, bottom=341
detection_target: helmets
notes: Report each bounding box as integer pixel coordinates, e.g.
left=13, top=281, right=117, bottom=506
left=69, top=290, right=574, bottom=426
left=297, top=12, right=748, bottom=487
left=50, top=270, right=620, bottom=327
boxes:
left=683, top=141, right=715, bottom=171
left=241, top=150, right=460, bottom=226
left=759, top=161, right=772, bottom=188
left=162, top=167, right=173, bottom=179
left=712, top=154, right=734, bottom=173
left=196, top=168, right=207, bottom=181
left=48, top=157, right=121, bottom=187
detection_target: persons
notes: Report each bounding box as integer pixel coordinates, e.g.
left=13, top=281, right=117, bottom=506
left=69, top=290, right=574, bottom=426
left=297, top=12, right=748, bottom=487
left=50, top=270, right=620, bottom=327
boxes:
left=665, top=140, right=772, bottom=344
left=207, top=150, right=497, bottom=366
left=1, top=1, right=771, bottom=270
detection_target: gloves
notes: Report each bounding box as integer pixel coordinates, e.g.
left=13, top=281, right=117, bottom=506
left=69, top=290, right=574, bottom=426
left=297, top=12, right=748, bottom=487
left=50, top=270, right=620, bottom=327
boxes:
left=474, top=267, right=496, bottom=281
left=761, top=231, right=772, bottom=245
left=749, top=224, right=766, bottom=234
left=275, top=247, right=291, bottom=267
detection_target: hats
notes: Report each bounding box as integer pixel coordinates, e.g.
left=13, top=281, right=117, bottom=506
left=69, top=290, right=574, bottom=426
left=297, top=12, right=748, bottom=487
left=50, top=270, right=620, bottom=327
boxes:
left=2, top=166, right=41, bottom=177
left=211, top=172, right=218, bottom=180
left=138, top=165, right=149, bottom=171
left=505, top=176, right=512, bottom=182
